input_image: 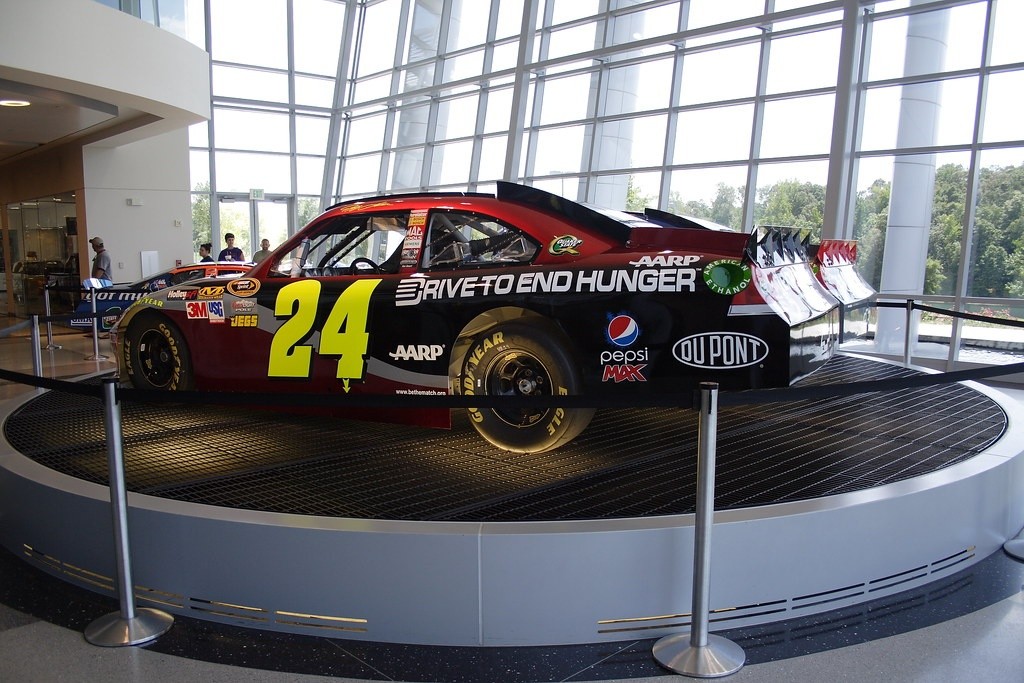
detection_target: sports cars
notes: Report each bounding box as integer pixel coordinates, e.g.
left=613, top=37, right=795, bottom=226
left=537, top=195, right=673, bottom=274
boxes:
left=71, top=260, right=257, bottom=333
left=107, top=180, right=878, bottom=458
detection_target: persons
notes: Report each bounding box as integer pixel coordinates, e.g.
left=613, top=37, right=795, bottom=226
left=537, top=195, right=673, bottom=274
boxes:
left=84, top=236, right=112, bottom=339
left=252, top=239, right=281, bottom=270
left=199, top=243, right=214, bottom=262
left=217, top=233, right=245, bottom=274
left=65, top=252, right=79, bottom=274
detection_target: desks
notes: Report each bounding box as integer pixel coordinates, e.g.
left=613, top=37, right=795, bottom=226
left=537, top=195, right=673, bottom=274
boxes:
left=22, top=274, right=81, bottom=310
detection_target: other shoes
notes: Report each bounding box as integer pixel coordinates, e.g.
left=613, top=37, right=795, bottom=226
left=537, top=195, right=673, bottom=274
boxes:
left=83, top=333, right=99, bottom=338
left=99, top=333, right=110, bottom=339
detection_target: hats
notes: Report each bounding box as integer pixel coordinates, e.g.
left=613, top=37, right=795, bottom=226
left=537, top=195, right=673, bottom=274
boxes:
left=89, top=237, right=104, bottom=247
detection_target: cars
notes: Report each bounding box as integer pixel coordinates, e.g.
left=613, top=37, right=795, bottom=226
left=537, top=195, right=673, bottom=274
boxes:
left=12, top=259, right=70, bottom=305
